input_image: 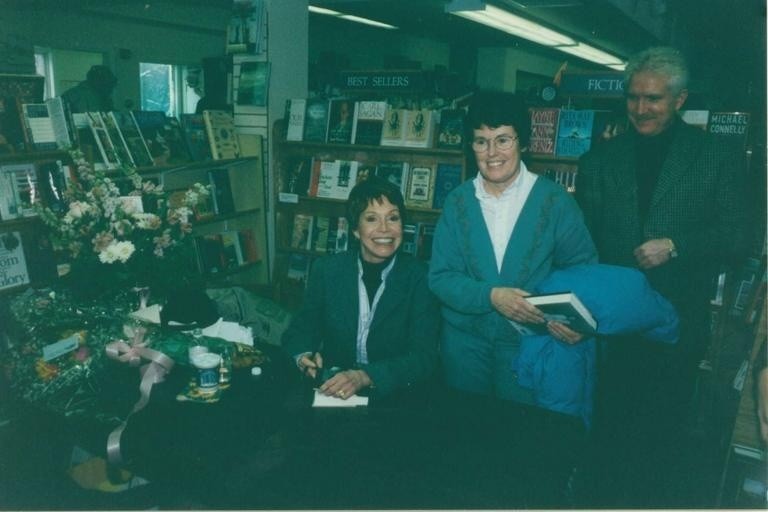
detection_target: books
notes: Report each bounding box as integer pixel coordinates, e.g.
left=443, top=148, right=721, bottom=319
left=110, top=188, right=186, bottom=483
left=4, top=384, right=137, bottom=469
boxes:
left=4, top=102, right=257, bottom=289
left=529, top=106, right=760, bottom=336
left=505, top=291, right=598, bottom=337
left=283, top=99, right=467, bottom=291
left=224, top=3, right=271, bottom=106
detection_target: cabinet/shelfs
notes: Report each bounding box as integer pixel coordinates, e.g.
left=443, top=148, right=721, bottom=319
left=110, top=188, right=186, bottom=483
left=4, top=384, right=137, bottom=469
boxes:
left=1, top=138, right=595, bottom=324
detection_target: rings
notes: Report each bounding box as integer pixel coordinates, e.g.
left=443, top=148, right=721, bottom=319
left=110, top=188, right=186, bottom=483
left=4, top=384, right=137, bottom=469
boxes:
left=337, top=390, right=345, bottom=396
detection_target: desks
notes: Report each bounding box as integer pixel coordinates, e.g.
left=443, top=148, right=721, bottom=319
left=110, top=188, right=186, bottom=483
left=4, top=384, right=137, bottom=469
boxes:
left=6, top=368, right=595, bottom=509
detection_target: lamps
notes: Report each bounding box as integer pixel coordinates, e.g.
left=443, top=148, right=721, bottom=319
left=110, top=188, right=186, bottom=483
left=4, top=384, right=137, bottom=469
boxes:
left=305, top=4, right=399, bottom=32
left=448, top=2, right=629, bottom=74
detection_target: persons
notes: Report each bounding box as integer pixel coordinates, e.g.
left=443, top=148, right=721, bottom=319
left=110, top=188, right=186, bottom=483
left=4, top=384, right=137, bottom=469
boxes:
left=279, top=174, right=443, bottom=399
left=748, top=334, right=768, bottom=444
left=572, top=42, right=750, bottom=440
left=330, top=101, right=352, bottom=144
left=63, top=65, right=117, bottom=114
left=428, top=87, right=600, bottom=425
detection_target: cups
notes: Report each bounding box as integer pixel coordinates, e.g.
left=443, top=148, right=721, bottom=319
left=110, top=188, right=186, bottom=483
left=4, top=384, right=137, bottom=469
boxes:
left=193, top=352, right=222, bottom=395
left=216, top=344, right=233, bottom=389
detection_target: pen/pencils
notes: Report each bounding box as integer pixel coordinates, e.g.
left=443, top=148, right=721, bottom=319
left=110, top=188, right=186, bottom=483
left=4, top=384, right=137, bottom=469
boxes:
left=300, top=339, right=323, bottom=381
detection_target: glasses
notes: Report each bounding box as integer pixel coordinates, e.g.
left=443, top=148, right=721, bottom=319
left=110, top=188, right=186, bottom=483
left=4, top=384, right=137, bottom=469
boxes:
left=471, top=134, right=520, bottom=152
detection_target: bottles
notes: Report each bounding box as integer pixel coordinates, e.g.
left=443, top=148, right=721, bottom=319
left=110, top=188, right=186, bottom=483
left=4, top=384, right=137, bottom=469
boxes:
left=187, top=328, right=210, bottom=382
